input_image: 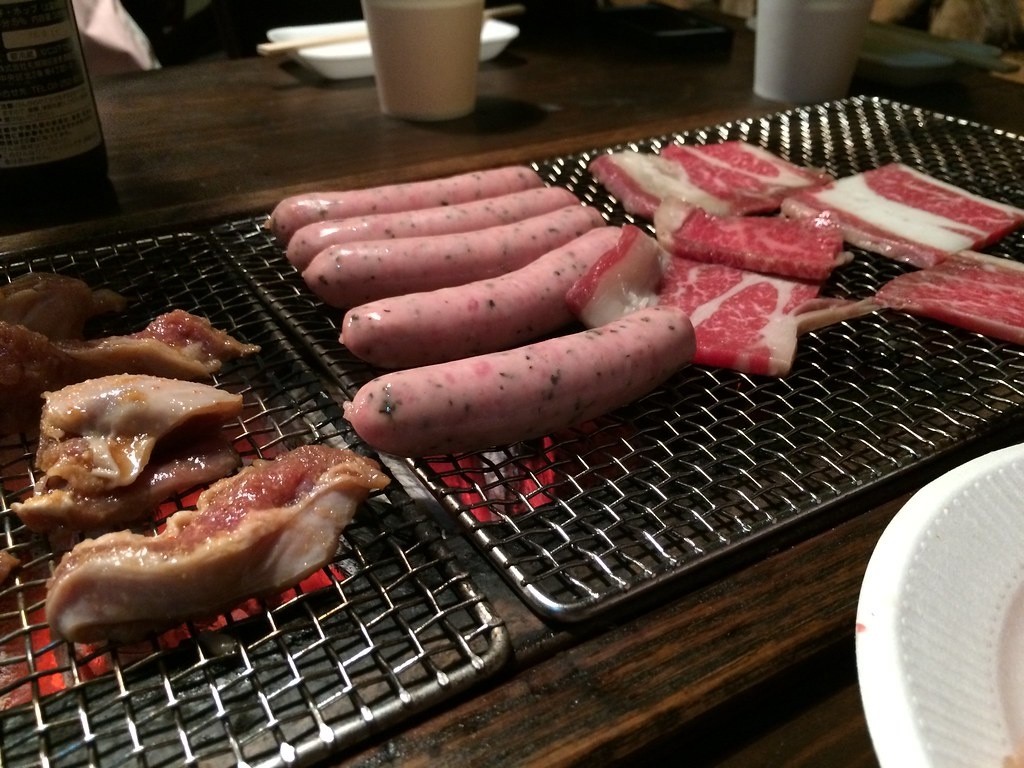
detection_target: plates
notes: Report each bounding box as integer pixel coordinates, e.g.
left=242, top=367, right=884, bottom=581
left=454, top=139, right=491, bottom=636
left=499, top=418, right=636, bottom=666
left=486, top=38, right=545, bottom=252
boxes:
left=267, top=15, right=519, bottom=80
left=855, top=441, right=1024, bottom=768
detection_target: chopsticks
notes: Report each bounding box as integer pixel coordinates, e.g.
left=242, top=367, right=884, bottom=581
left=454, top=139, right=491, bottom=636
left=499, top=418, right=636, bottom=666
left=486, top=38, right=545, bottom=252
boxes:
left=258, top=5, right=523, bottom=56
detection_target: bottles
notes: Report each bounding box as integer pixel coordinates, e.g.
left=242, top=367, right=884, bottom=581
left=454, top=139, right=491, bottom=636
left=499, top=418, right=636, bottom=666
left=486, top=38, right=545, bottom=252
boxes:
left=0, top=0, right=108, bottom=227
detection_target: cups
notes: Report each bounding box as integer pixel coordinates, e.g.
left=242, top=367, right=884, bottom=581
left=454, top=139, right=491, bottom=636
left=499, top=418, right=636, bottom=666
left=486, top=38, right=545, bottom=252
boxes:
left=752, top=0, right=872, bottom=104
left=362, top=0, right=484, bottom=119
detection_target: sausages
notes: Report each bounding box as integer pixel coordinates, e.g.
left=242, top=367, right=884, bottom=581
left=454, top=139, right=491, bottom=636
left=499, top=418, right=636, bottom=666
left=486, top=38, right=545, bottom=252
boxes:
left=263, top=164, right=695, bottom=455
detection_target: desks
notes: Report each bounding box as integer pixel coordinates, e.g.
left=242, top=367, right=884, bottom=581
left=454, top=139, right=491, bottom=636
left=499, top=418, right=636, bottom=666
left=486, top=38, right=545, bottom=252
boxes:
left=0, top=0, right=1024, bottom=254
